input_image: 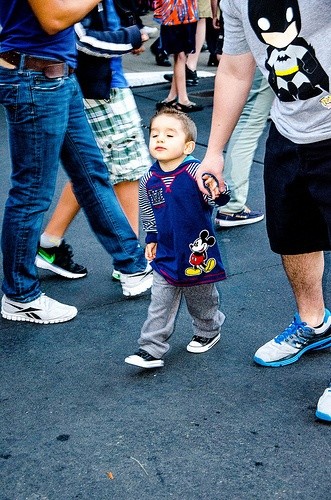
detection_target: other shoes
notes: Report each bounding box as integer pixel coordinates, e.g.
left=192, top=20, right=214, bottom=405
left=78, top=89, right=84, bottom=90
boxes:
left=151, top=48, right=171, bottom=66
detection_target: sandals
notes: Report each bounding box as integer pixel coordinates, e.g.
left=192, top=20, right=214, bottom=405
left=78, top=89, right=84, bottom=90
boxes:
left=156, top=99, right=176, bottom=109
left=175, top=101, right=205, bottom=112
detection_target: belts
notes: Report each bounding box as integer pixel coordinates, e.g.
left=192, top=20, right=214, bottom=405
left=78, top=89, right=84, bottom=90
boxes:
left=0, top=51, right=75, bottom=78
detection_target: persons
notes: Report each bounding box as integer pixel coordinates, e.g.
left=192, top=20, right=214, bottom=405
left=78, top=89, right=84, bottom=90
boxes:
left=0, top=0, right=274, bottom=325
left=195, top=0, right=331, bottom=421
left=125, top=108, right=231, bottom=369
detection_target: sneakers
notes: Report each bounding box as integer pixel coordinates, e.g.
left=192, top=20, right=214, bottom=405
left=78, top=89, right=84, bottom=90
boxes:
left=34, top=238, right=88, bottom=278
left=120, top=258, right=154, bottom=296
left=139, top=25, right=158, bottom=41
left=124, top=350, right=164, bottom=368
left=186, top=332, right=221, bottom=353
left=164, top=67, right=199, bottom=86
left=111, top=269, right=120, bottom=280
left=1, top=293, right=77, bottom=324
left=315, top=387, right=331, bottom=421
left=253, top=309, right=331, bottom=367
left=216, top=206, right=264, bottom=227
